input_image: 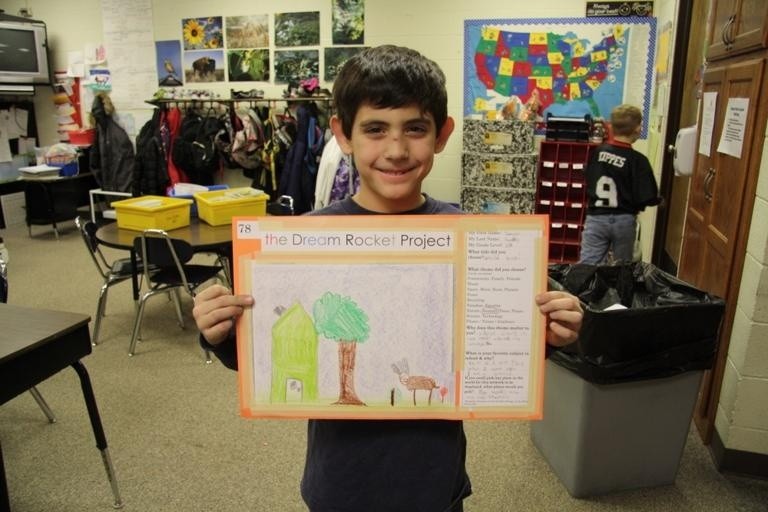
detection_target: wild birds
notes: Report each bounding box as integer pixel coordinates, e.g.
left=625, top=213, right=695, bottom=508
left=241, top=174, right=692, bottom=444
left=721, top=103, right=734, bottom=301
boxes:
left=163, top=57, right=178, bottom=77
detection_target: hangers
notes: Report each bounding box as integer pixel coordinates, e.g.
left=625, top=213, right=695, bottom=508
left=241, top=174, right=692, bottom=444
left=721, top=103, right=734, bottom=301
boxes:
left=160, top=101, right=317, bottom=129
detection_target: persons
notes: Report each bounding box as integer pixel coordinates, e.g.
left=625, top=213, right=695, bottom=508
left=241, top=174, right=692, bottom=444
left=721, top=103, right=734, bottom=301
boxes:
left=190, top=42, right=584, bottom=512
left=578, top=102, right=666, bottom=263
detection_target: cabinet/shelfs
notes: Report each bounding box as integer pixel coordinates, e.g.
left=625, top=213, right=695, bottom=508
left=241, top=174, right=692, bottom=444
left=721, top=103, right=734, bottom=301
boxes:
left=460, top=115, right=600, bottom=264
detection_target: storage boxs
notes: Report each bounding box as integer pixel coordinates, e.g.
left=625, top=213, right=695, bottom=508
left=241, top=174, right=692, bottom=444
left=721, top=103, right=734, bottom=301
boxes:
left=168, top=184, right=270, bottom=227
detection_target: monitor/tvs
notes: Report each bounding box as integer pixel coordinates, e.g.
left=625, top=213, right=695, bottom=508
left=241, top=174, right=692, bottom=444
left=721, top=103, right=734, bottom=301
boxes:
left=0, top=13, right=51, bottom=86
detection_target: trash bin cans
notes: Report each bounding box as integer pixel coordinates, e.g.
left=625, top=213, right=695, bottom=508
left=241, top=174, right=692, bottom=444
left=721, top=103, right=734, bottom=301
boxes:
left=530, top=261, right=727, bottom=498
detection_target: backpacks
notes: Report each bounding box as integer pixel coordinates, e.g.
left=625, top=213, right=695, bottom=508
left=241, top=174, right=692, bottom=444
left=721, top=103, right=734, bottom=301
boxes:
left=174, top=102, right=328, bottom=217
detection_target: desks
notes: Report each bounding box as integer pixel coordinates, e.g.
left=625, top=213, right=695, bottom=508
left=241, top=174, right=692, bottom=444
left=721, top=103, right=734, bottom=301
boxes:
left=16, top=172, right=105, bottom=241
left=0, top=300, right=124, bottom=511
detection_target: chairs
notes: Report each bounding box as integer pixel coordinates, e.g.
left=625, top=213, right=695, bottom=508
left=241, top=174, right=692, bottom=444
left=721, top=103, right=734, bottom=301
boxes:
left=74, top=195, right=295, bottom=364
